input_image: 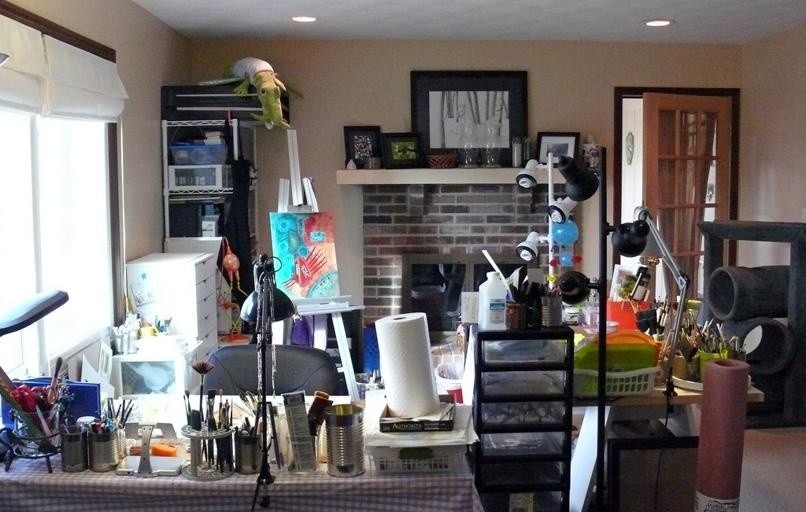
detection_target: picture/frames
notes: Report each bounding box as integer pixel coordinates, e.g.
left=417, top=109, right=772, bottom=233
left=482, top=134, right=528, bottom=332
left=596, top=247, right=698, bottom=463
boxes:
left=343, top=126, right=426, bottom=169
left=535, top=133, right=578, bottom=166
left=411, top=70, right=527, bottom=168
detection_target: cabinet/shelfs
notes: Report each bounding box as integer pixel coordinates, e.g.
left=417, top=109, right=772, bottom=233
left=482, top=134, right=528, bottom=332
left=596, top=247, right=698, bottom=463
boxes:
left=161, top=119, right=260, bottom=336
left=461, top=323, right=573, bottom=512
left=127, top=252, right=219, bottom=364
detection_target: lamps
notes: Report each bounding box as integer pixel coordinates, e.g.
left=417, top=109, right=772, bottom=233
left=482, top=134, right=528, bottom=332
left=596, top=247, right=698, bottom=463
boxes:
left=240, top=254, right=296, bottom=485
left=1, top=289, right=101, bottom=430
left=516, top=151, right=652, bottom=511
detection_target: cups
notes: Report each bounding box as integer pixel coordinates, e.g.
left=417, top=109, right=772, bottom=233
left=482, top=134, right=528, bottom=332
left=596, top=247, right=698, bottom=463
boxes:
left=505, top=301, right=563, bottom=330
left=673, top=347, right=746, bottom=384
left=285, top=434, right=320, bottom=476
left=114, top=326, right=168, bottom=354
left=326, top=403, right=365, bottom=477
left=234, top=431, right=263, bottom=474
left=14, top=409, right=126, bottom=472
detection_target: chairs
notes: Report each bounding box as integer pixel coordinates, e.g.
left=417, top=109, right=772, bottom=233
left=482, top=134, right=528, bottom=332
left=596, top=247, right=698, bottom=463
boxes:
left=200, top=345, right=337, bottom=396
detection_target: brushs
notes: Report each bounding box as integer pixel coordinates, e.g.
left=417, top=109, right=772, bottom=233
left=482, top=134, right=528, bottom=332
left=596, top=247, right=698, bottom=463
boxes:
left=287, top=391, right=333, bottom=471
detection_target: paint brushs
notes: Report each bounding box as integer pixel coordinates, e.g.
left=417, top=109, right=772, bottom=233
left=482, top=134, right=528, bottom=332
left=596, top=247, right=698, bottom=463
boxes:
left=108, top=398, right=134, bottom=428
left=0, top=358, right=76, bottom=444
left=183, top=362, right=258, bottom=472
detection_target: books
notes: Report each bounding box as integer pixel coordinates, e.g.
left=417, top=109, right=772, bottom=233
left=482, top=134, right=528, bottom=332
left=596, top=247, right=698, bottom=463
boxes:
left=202, top=130, right=226, bottom=146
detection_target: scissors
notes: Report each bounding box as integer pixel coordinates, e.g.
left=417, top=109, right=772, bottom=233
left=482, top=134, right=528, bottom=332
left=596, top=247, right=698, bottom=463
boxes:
left=11, top=384, right=56, bottom=435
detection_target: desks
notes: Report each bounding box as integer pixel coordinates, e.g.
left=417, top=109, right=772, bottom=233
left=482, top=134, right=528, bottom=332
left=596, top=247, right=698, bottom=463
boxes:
left=1, top=395, right=474, bottom=512
left=572, top=372, right=764, bottom=512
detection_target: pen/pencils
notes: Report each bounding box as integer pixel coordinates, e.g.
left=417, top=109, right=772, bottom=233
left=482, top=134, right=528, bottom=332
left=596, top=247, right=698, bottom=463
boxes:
left=509, top=280, right=562, bottom=306
left=86, top=408, right=118, bottom=434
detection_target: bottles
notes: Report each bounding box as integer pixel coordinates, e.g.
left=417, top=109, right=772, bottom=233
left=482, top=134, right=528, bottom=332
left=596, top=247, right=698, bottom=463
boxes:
left=460, top=119, right=534, bottom=169
left=478, top=271, right=507, bottom=332
